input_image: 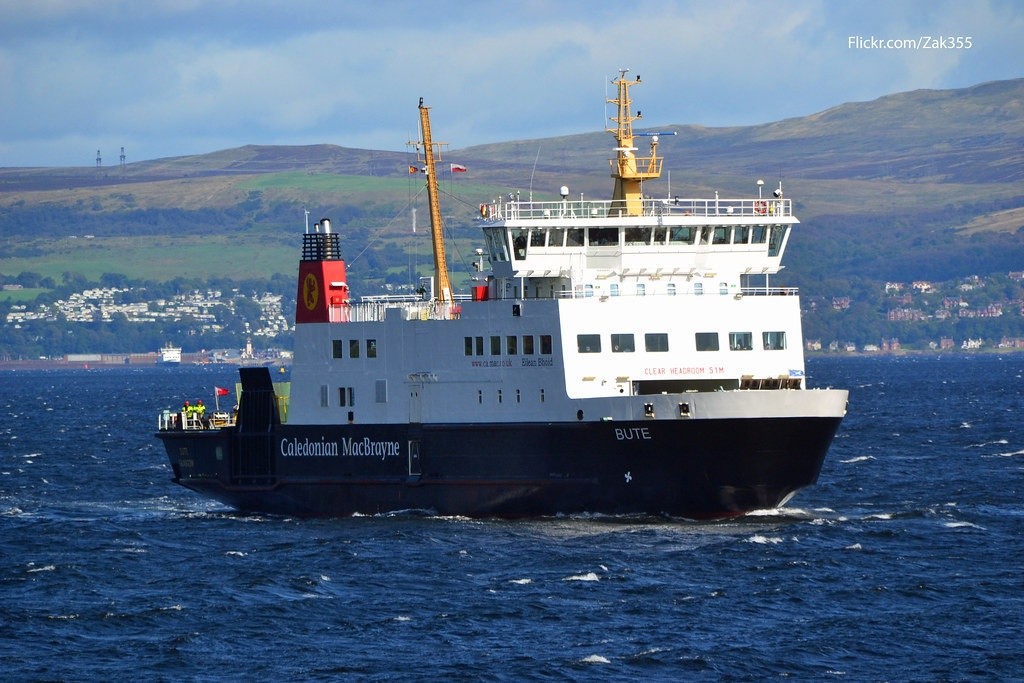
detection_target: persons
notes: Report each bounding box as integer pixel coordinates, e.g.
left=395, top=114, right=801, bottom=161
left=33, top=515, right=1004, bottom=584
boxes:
left=182, top=401, right=193, bottom=426
left=193, top=400, right=206, bottom=425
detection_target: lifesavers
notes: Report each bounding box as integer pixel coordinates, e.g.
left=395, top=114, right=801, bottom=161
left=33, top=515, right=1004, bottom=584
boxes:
left=755, top=202, right=766, bottom=213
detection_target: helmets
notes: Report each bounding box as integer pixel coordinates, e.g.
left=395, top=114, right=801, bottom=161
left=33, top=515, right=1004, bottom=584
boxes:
left=185, top=401, right=190, bottom=406
left=198, top=400, right=203, bottom=405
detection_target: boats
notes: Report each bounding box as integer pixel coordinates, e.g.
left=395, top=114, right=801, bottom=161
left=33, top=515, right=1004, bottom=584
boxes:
left=156, top=67, right=851, bottom=525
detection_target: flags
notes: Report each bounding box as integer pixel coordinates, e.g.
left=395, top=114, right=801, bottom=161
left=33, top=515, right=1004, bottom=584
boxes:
left=217, top=388, right=229, bottom=396
left=410, top=166, right=419, bottom=176
left=451, top=164, right=467, bottom=172
